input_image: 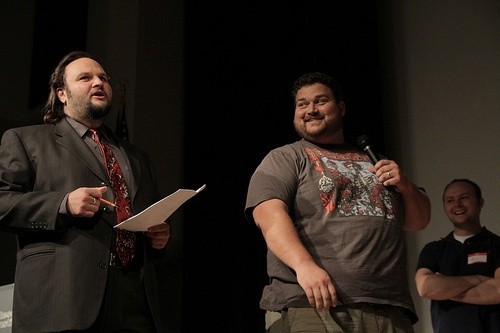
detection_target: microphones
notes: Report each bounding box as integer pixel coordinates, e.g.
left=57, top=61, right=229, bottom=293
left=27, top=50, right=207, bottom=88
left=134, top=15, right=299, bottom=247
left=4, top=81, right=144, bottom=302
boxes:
left=357, top=135, right=395, bottom=192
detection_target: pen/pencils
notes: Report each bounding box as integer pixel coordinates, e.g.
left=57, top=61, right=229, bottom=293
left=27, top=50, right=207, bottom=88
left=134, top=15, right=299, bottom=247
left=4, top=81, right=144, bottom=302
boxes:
left=97, top=197, right=119, bottom=209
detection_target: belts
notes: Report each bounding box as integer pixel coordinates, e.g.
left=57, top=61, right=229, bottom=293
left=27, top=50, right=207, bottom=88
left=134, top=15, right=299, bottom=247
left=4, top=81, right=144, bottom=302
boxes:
left=107, top=252, right=124, bottom=269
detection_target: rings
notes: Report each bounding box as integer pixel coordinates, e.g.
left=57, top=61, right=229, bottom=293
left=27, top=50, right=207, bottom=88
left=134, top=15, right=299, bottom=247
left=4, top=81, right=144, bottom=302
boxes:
left=92, top=197, right=98, bottom=205
left=384, top=169, right=393, bottom=178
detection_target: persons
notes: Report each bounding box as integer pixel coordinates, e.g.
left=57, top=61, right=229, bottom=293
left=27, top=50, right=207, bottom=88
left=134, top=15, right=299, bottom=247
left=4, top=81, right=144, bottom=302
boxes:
left=413, top=178, right=500, bottom=332
left=0, top=53, right=171, bottom=333
left=243, top=72, right=431, bottom=333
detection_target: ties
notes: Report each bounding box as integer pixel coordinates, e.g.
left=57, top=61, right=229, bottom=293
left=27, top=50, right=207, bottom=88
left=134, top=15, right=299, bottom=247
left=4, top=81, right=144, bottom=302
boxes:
left=87, top=128, right=137, bottom=268
